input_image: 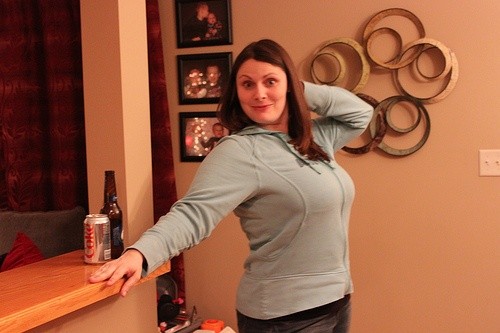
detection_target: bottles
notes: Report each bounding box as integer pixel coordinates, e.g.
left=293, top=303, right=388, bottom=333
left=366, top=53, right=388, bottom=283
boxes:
left=100, top=171, right=124, bottom=259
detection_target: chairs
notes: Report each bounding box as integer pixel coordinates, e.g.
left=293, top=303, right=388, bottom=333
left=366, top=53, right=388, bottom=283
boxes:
left=156, top=275, right=178, bottom=301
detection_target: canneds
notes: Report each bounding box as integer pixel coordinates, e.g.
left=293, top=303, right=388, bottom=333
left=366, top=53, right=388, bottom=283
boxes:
left=83, top=213, right=111, bottom=264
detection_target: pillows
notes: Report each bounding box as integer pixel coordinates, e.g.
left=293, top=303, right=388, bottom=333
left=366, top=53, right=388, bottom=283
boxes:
left=0, top=231, right=47, bottom=272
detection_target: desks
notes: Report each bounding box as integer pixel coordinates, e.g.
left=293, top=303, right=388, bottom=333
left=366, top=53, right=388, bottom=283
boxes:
left=157, top=309, right=203, bottom=333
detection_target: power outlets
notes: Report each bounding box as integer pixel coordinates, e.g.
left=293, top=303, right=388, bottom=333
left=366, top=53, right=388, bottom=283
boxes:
left=480, top=150, right=500, bottom=176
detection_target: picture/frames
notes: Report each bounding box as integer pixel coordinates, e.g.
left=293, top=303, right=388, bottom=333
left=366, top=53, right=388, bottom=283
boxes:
left=179, top=111, right=232, bottom=162
left=177, top=51, right=234, bottom=104
left=175, top=0, right=233, bottom=49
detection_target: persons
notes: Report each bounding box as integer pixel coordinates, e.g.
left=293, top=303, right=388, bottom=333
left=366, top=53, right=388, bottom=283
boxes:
left=183, top=65, right=224, bottom=98
left=188, top=5, right=221, bottom=42
left=198, top=123, right=225, bottom=155
left=88, top=38, right=373, bottom=333
